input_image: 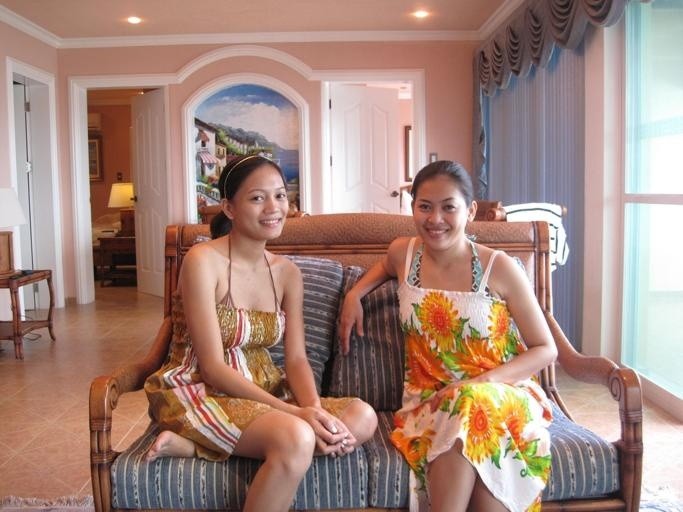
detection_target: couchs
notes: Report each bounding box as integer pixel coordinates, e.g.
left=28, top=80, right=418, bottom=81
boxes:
left=90, top=213, right=642, bottom=512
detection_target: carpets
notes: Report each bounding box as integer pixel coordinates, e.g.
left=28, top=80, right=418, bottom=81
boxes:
left=0, top=487, right=681, bottom=511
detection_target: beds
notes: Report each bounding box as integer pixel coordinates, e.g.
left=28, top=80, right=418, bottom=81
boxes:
left=92, top=213, right=122, bottom=266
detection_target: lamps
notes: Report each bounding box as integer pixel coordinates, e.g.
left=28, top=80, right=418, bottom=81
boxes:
left=0, top=187, right=27, bottom=275
left=108, top=182, right=134, bottom=236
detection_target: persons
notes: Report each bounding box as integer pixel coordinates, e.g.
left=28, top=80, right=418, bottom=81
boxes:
left=337, top=159, right=560, bottom=512
left=142, top=154, right=380, bottom=512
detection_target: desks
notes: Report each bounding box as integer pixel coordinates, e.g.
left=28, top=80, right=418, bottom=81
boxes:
left=97, top=237, right=137, bottom=287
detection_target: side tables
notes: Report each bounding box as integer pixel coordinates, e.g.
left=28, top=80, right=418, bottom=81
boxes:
left=0, top=270, right=56, bottom=361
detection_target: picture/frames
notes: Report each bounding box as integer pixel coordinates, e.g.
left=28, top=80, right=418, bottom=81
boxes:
left=89, top=135, right=104, bottom=182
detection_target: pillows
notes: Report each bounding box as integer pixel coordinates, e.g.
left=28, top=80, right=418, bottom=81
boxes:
left=327, top=265, right=406, bottom=410
left=193, top=235, right=344, bottom=398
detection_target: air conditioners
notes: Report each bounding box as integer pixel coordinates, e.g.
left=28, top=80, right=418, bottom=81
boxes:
left=88, top=112, right=101, bottom=132
